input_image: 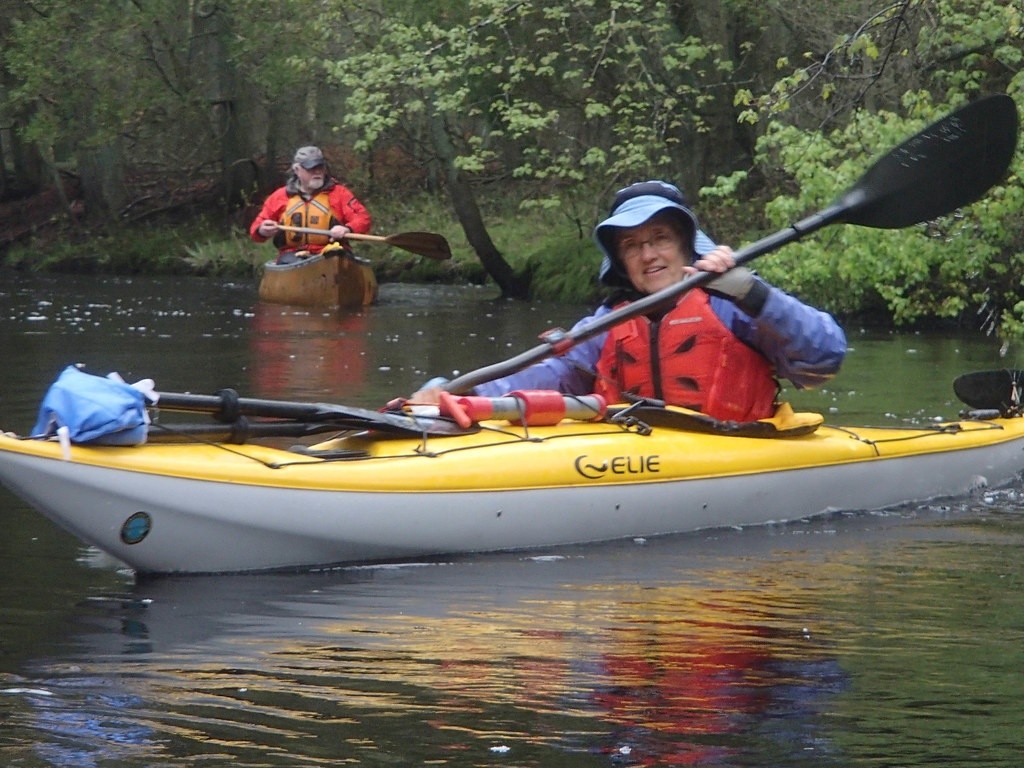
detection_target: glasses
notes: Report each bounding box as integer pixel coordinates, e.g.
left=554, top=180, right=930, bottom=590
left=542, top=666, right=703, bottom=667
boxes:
left=617, top=233, right=679, bottom=260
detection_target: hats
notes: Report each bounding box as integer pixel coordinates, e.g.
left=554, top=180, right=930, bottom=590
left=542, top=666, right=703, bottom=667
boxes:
left=593, top=180, right=718, bottom=287
left=295, top=145, right=325, bottom=170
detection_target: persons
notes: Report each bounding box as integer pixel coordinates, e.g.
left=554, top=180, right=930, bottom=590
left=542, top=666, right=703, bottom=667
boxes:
left=249, top=146, right=371, bottom=266
left=409, top=180, right=846, bottom=418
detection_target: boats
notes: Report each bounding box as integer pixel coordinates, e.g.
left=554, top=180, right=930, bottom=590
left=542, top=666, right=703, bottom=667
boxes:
left=257, top=247, right=379, bottom=309
left=1, top=401, right=1024, bottom=580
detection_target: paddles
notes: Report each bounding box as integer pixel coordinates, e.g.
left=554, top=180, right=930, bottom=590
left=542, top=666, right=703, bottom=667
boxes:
left=133, top=383, right=484, bottom=440
left=274, top=224, right=452, bottom=260
left=439, top=95, right=1021, bottom=395
